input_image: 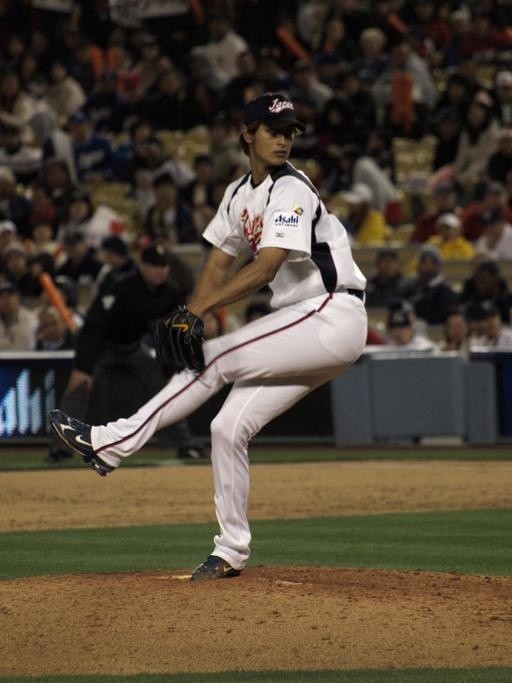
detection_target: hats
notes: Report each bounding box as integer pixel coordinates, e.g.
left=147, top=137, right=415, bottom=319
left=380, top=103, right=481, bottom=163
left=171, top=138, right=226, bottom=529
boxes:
left=3, top=242, right=25, bottom=259
left=474, top=301, right=502, bottom=319
left=436, top=211, right=463, bottom=229
left=386, top=309, right=414, bottom=328
left=0, top=220, right=16, bottom=235
left=237, top=93, right=310, bottom=135
left=138, top=241, right=176, bottom=267
left=342, top=183, right=377, bottom=205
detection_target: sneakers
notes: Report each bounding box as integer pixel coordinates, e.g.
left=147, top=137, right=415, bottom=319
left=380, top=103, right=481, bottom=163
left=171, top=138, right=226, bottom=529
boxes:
left=177, top=442, right=211, bottom=466
left=47, top=408, right=120, bottom=478
left=188, top=545, right=255, bottom=587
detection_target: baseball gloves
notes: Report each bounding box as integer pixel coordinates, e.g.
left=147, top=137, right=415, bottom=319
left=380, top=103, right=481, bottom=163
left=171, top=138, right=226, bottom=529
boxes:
left=151, top=302, right=206, bottom=373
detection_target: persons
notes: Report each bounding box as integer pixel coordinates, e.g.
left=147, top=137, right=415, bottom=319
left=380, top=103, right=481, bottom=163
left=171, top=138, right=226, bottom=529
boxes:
left=44, top=244, right=207, bottom=464
left=50, top=91, right=369, bottom=583
left=1, top=2, right=512, bottom=351
